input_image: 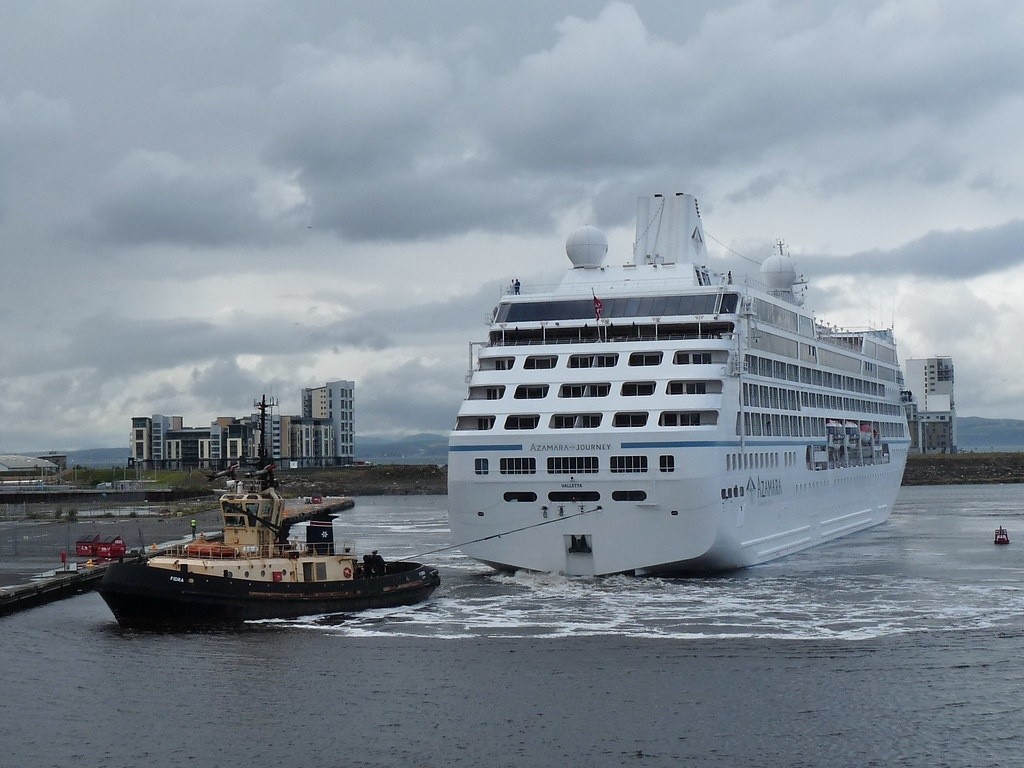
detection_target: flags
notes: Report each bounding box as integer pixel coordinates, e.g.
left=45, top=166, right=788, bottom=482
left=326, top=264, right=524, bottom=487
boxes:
left=594, top=297, right=603, bottom=321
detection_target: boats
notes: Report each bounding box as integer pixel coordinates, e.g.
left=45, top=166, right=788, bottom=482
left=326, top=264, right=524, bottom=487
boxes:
left=98, top=394, right=443, bottom=633
left=993, top=524, right=1009, bottom=544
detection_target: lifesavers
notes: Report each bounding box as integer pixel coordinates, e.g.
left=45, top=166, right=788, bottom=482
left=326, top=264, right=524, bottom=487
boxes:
left=343, top=567, right=352, bottom=579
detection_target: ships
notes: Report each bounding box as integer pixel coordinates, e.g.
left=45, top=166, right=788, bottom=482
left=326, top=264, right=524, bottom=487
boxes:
left=447, top=193, right=910, bottom=583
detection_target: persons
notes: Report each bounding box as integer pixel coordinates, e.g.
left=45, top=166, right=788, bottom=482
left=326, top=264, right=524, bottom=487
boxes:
left=121, top=484, right=123, bottom=490
left=510, top=279, right=520, bottom=295
left=372, top=550, right=383, bottom=578
left=728, top=271, right=732, bottom=285
left=191, top=517, right=196, bottom=538
